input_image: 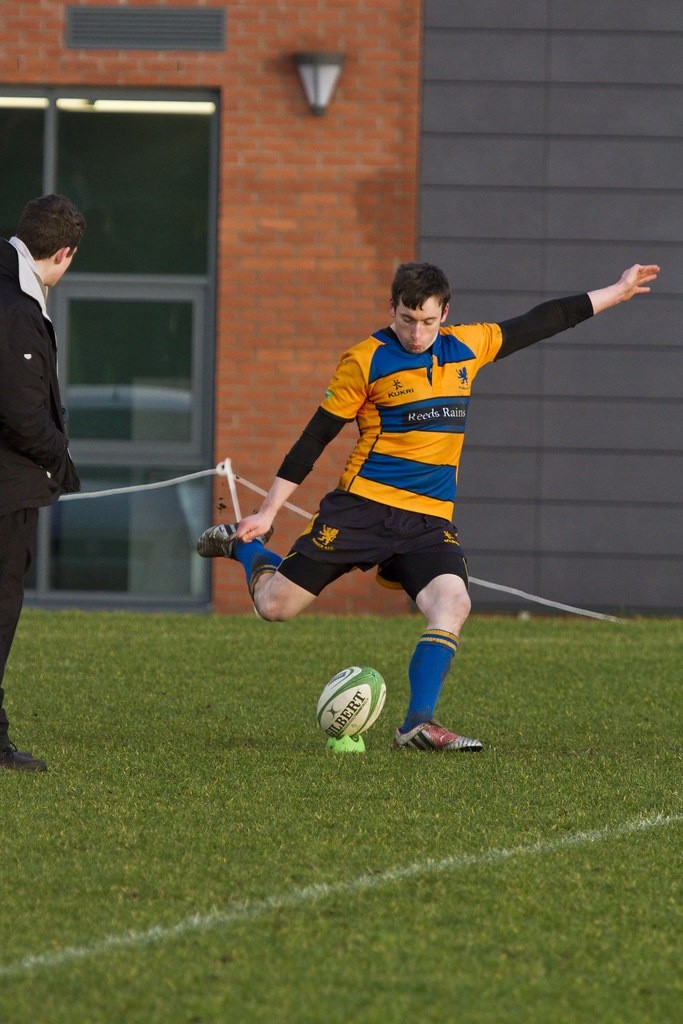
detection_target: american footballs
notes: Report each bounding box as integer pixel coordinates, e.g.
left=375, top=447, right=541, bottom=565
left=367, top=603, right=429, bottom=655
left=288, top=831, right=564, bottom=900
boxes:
left=314, top=662, right=391, bottom=738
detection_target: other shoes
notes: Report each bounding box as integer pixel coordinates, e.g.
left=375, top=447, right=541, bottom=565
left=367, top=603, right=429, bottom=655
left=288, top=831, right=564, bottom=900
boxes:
left=0, top=741, right=48, bottom=773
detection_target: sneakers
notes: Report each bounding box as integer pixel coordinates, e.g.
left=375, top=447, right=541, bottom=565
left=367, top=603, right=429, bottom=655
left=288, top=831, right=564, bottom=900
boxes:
left=393, top=721, right=483, bottom=752
left=196, top=522, right=273, bottom=558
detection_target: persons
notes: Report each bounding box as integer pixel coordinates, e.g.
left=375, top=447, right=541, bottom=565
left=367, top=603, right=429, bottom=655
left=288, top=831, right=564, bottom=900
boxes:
left=198, top=263, right=660, bottom=751
left=0, top=194, right=84, bottom=771
